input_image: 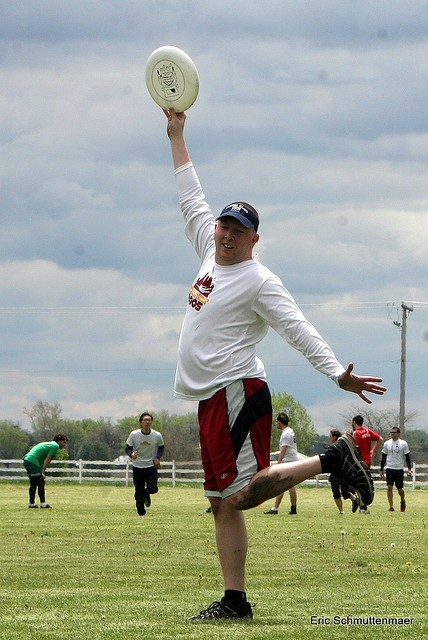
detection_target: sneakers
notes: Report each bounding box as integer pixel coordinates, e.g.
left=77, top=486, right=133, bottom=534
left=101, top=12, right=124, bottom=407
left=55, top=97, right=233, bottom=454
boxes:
left=323, top=432, right=374, bottom=506
left=287, top=511, right=298, bottom=515
left=400, top=499, right=406, bottom=512
left=187, top=596, right=254, bottom=621
left=29, top=503, right=39, bottom=508
left=390, top=507, right=394, bottom=511
left=136, top=505, right=146, bottom=516
left=352, top=495, right=360, bottom=513
left=264, top=509, right=278, bottom=515
left=205, top=504, right=212, bottom=512
left=144, top=487, right=151, bottom=507
left=41, top=503, right=52, bottom=508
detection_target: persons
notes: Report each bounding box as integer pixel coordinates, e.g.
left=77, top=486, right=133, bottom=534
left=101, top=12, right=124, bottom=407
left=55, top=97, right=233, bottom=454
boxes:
left=123, top=412, right=165, bottom=518
left=328, top=425, right=359, bottom=516
left=20, top=434, right=69, bottom=510
left=350, top=414, right=384, bottom=515
left=161, top=106, right=388, bottom=621
left=378, top=427, right=414, bottom=513
left=262, top=412, right=298, bottom=515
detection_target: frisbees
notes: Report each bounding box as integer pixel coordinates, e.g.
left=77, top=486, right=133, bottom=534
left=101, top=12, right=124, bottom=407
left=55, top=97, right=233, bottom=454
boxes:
left=145, top=46, right=199, bottom=113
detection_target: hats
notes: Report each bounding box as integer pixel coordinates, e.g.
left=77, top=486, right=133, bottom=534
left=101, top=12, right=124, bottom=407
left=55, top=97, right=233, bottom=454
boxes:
left=277, top=413, right=289, bottom=425
left=214, top=203, right=259, bottom=232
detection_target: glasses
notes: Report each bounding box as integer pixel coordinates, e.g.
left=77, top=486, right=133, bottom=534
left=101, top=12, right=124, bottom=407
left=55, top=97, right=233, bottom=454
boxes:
left=390, top=432, right=397, bottom=433
